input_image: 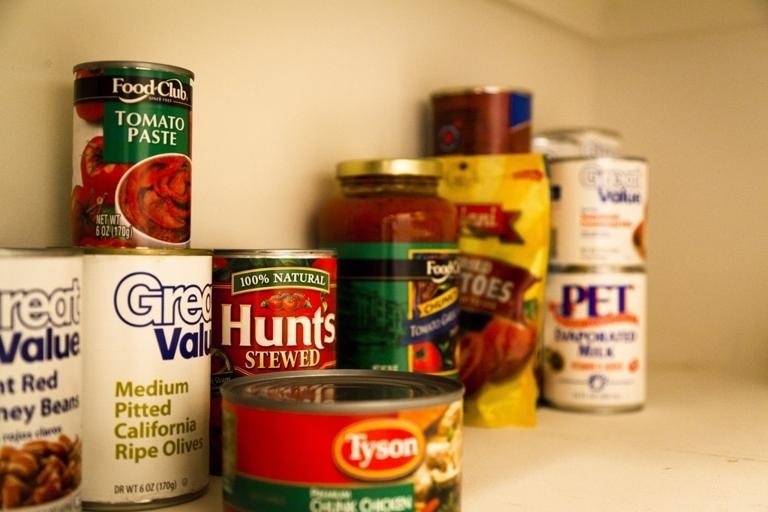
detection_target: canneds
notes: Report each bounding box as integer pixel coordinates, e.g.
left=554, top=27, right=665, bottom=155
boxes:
left=71, top=59, right=195, bottom=251
left=0, top=246, right=82, bottom=512
left=538, top=268, right=648, bottom=416
left=83, top=248, right=215, bottom=512
left=317, top=158, right=462, bottom=386
left=546, top=154, right=649, bottom=268
left=220, top=368, right=467, bottom=512
left=212, top=248, right=340, bottom=480
left=430, top=86, right=534, bottom=157
